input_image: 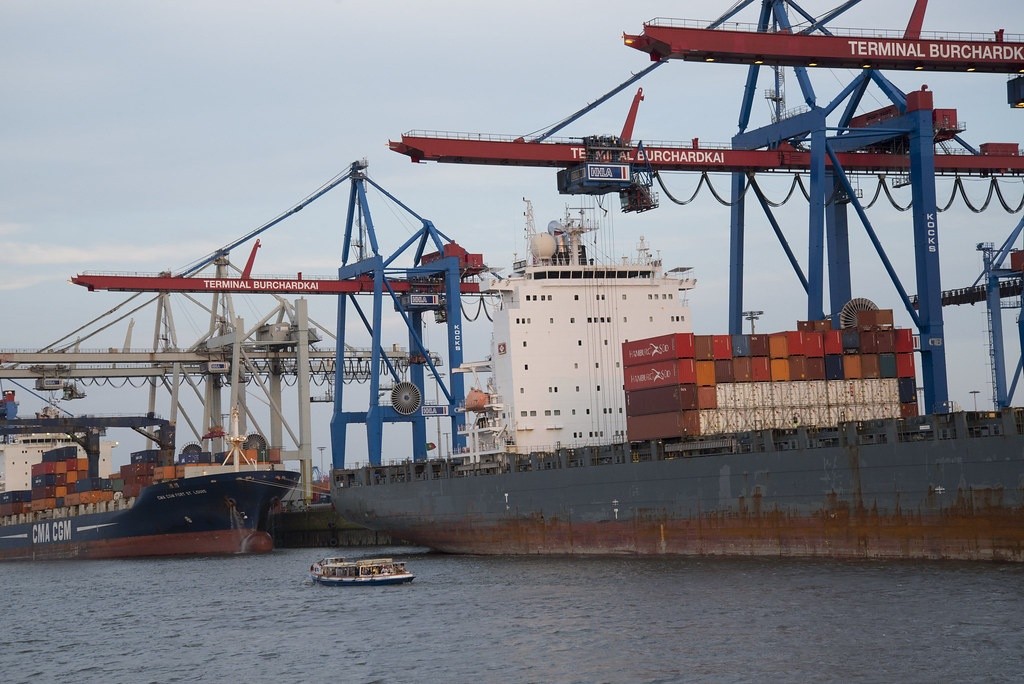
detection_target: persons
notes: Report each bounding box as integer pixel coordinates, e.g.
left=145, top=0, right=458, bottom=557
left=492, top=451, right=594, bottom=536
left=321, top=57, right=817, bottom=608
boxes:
left=368, top=564, right=408, bottom=574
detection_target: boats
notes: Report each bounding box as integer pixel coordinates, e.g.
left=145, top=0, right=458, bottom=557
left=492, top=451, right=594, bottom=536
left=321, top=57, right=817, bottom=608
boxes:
left=310, top=556, right=418, bottom=587
left=326, top=1, right=1024, bottom=571
left=0, top=387, right=302, bottom=558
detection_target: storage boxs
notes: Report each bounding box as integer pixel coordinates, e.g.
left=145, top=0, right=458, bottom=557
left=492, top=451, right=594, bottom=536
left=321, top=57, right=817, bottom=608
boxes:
left=620, top=308, right=920, bottom=442
left=0, top=445, right=281, bottom=520
